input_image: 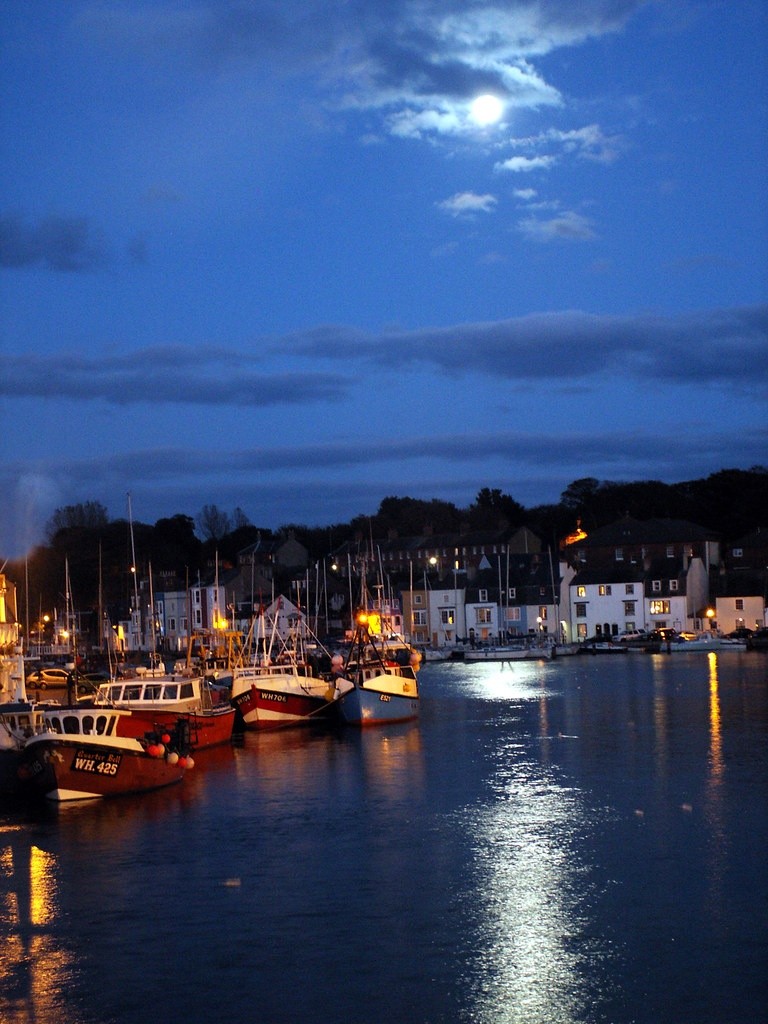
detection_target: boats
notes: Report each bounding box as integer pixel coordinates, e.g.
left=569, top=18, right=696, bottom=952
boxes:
left=641, top=598, right=722, bottom=654
left=720, top=638, right=747, bottom=653
left=580, top=640, right=629, bottom=655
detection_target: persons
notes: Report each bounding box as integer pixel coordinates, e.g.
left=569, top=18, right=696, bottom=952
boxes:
left=395, top=650, right=411, bottom=676
left=383, top=650, right=397, bottom=662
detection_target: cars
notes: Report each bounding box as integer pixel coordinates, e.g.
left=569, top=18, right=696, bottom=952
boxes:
left=25, top=667, right=82, bottom=690
left=649, top=627, right=678, bottom=643
left=721, top=626, right=755, bottom=639
left=678, top=631, right=697, bottom=641
left=745, top=627, right=768, bottom=650
left=72, top=672, right=112, bottom=694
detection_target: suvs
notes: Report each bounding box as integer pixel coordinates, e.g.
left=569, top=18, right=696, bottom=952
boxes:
left=611, top=628, right=648, bottom=643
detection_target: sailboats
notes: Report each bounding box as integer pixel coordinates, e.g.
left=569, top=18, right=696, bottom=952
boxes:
left=0, top=490, right=582, bottom=805
